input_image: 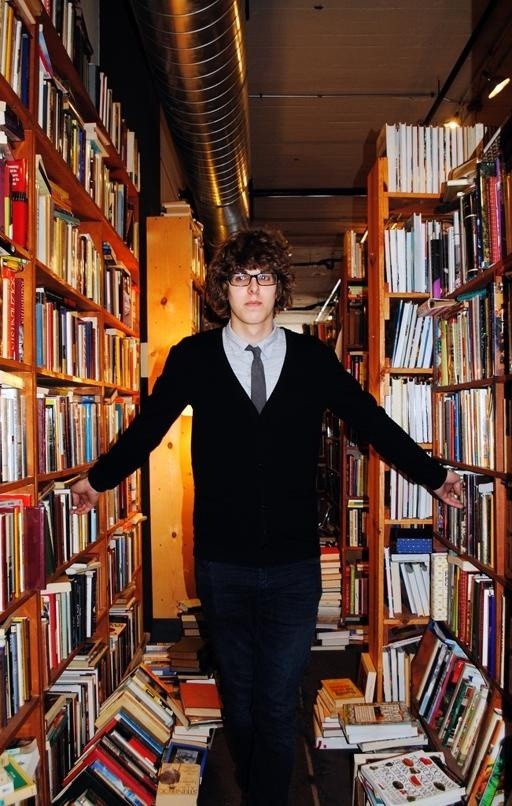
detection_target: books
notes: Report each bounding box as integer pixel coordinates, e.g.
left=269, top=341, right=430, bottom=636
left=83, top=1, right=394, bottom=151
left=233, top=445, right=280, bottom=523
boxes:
left=310, top=121, right=511, bottom=805
left=0, top=0, right=225, bottom=806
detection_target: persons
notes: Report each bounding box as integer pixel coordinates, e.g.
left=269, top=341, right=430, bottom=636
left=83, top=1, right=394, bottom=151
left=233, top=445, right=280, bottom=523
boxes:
left=69, top=228, right=464, bottom=806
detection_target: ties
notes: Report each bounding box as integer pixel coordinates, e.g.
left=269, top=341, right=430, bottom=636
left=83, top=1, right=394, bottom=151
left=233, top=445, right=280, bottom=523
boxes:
left=245, top=344, right=266, bottom=415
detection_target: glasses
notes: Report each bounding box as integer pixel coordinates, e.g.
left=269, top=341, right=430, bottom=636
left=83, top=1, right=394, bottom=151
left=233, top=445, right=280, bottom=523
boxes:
left=221, top=271, right=281, bottom=286
